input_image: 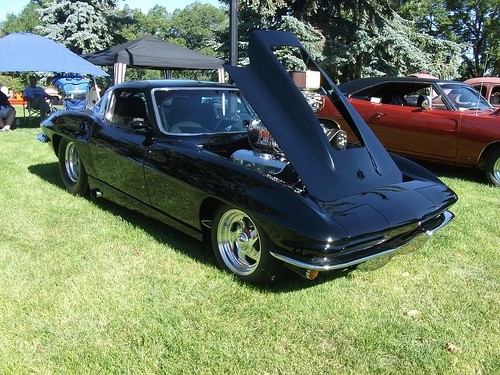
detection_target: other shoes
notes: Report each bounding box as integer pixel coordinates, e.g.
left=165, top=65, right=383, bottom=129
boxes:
left=1, top=126, right=12, bottom=132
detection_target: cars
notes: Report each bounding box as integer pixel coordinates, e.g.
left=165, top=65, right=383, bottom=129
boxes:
left=311, top=72, right=500, bottom=186
left=36, top=29, right=458, bottom=283
left=463, top=77, right=500, bottom=112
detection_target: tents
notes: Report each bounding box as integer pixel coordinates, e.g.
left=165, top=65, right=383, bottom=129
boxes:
left=80, top=35, right=227, bottom=116
left=0, top=32, right=109, bottom=101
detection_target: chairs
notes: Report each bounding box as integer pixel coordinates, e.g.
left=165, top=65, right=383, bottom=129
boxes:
left=21, top=91, right=51, bottom=124
left=392, top=93, right=407, bottom=105
left=124, top=97, right=218, bottom=124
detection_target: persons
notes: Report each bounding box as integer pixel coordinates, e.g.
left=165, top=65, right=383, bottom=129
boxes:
left=0, top=91, right=16, bottom=132
left=22, top=75, right=53, bottom=123
